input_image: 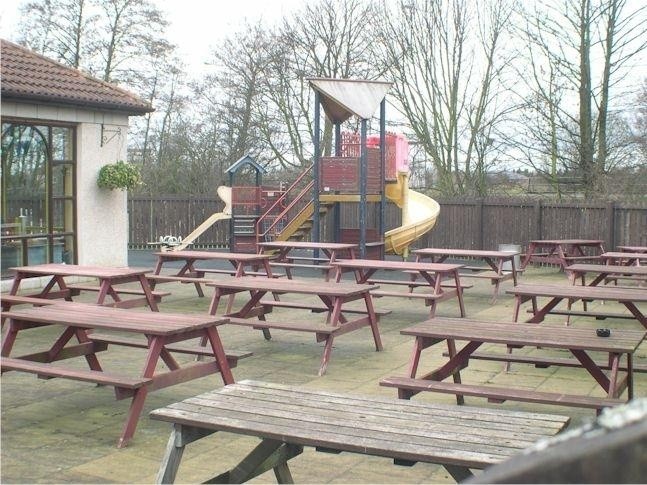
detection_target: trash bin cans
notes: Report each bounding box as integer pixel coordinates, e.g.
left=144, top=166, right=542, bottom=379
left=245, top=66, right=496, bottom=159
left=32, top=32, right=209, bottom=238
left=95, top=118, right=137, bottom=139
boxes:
left=498, top=243, right=521, bottom=275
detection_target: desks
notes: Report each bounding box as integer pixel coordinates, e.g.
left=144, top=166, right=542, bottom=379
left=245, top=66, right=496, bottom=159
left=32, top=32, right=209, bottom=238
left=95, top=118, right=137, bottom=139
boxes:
left=151, top=249, right=277, bottom=269
left=503, top=281, right=647, bottom=311
left=564, top=262, right=647, bottom=281
left=254, top=239, right=360, bottom=255
left=527, top=236, right=602, bottom=248
left=616, top=244, right=647, bottom=252
left=2, top=301, right=233, bottom=343
left=600, top=250, right=647, bottom=263
left=410, top=246, right=522, bottom=263
left=148, top=375, right=574, bottom=484
left=329, top=258, right=466, bottom=276
left=7, top=262, right=154, bottom=286
left=194, top=278, right=385, bottom=378
left=398, top=315, right=646, bottom=360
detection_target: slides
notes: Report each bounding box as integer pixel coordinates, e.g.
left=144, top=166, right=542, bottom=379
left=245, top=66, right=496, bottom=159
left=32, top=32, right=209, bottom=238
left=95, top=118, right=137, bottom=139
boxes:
left=384, top=177, right=440, bottom=255
left=172, top=213, right=232, bottom=251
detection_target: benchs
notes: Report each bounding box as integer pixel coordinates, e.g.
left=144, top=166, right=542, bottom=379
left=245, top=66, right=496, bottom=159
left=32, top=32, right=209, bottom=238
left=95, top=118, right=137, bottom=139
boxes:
left=269, top=261, right=335, bottom=276
left=462, top=263, right=527, bottom=282
left=366, top=278, right=475, bottom=293
left=65, top=283, right=171, bottom=312
left=145, top=273, right=215, bottom=290
left=0, top=292, right=54, bottom=330
left=440, top=346, right=647, bottom=377
left=1, top=353, right=156, bottom=397
left=368, top=287, right=447, bottom=313
left=193, top=268, right=286, bottom=279
left=288, top=255, right=335, bottom=264
left=519, top=249, right=603, bottom=263
left=260, top=296, right=393, bottom=322
left=402, top=269, right=507, bottom=290
left=83, top=332, right=253, bottom=387
left=376, top=373, right=632, bottom=418
left=601, top=260, right=647, bottom=283
left=217, top=314, right=343, bottom=338
